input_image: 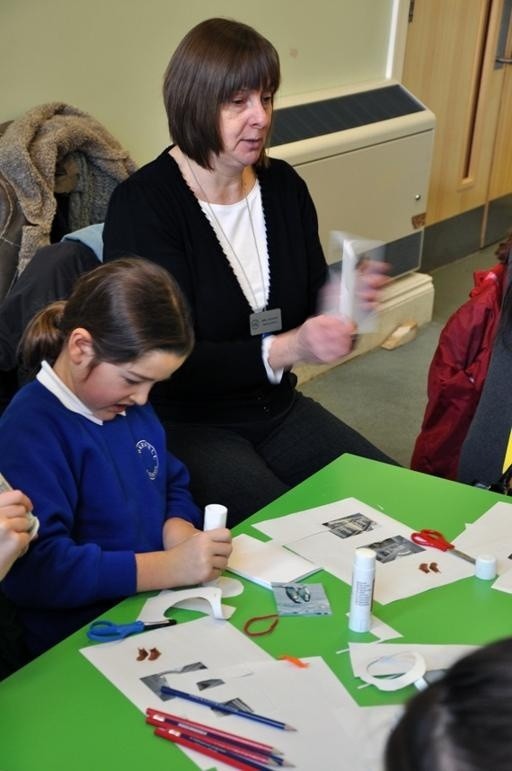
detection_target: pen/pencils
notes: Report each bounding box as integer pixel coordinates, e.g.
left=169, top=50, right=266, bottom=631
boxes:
left=145, top=708, right=297, bottom=771
left=160, top=686, right=298, bottom=731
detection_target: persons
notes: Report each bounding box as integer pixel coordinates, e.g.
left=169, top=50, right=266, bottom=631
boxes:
left=102, top=17, right=405, bottom=532
left=1, top=256, right=233, bottom=650
left=0, top=489, right=34, bottom=585
left=383, top=634, right=512, bottom=771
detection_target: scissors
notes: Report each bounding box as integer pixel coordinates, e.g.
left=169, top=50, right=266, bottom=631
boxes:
left=87, top=617, right=176, bottom=642
left=412, top=529, right=476, bottom=564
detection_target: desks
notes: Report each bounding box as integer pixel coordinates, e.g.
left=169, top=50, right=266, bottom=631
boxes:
left=0, top=453, right=512, bottom=771
